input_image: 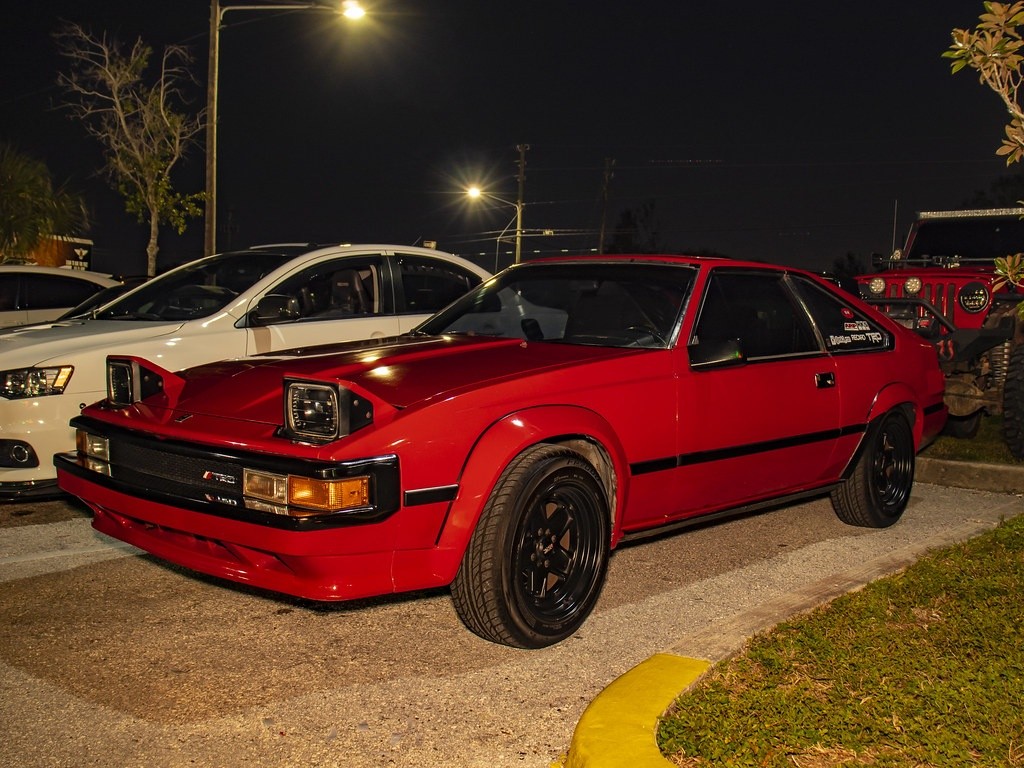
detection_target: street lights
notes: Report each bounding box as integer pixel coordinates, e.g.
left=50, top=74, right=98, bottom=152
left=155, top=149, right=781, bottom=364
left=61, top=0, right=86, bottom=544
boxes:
left=467, top=186, right=526, bottom=265
left=202, top=1, right=369, bottom=313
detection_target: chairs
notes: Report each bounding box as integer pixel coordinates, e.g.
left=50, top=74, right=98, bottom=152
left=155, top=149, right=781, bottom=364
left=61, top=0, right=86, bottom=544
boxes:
left=311, top=268, right=369, bottom=318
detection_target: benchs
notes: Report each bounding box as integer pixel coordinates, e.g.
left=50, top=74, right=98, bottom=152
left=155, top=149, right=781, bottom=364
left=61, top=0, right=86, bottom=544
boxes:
left=747, top=311, right=811, bottom=356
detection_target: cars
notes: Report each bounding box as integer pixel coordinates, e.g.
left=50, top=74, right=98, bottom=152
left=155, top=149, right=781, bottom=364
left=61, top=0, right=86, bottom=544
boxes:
left=0, top=263, right=127, bottom=334
left=0, top=242, right=571, bottom=508
left=52, top=249, right=949, bottom=653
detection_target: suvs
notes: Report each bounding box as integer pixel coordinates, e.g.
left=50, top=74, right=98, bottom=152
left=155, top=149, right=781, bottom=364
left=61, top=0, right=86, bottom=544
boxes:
left=826, top=205, right=1024, bottom=466
